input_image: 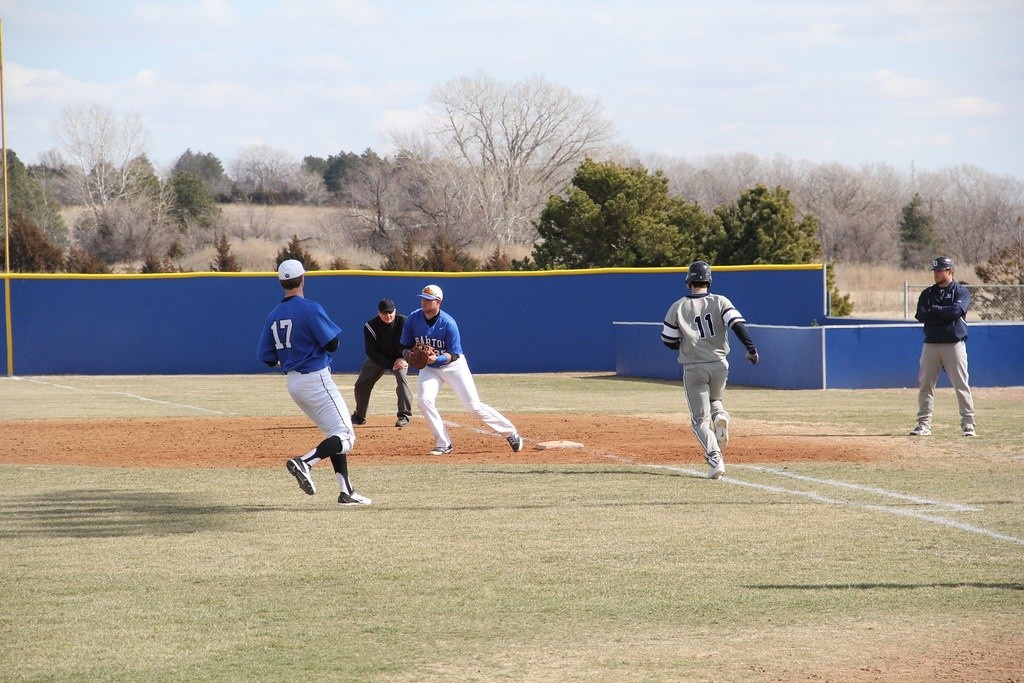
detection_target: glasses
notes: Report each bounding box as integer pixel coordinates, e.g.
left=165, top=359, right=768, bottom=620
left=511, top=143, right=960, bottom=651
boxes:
left=381, top=311, right=393, bottom=314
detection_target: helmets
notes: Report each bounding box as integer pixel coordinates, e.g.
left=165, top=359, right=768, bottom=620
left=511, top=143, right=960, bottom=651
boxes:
left=931, top=256, right=954, bottom=271
left=686, top=261, right=712, bottom=288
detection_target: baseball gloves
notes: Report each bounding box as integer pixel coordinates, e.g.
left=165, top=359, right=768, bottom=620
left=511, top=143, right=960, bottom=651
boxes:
left=405, top=341, right=435, bottom=370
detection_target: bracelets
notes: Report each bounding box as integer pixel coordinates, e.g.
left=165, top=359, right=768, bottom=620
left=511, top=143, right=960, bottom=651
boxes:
left=404, top=350, right=408, bottom=357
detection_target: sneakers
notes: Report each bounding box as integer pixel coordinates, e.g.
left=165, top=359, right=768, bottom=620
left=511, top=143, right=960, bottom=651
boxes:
left=286, top=457, right=316, bottom=495
left=707, top=462, right=725, bottom=479
left=713, top=414, right=729, bottom=451
left=962, top=427, right=975, bottom=436
left=507, top=432, right=522, bottom=451
left=337, top=490, right=372, bottom=506
left=430, top=444, right=453, bottom=455
left=910, top=425, right=931, bottom=436
left=350, top=411, right=366, bottom=427
left=395, top=413, right=411, bottom=427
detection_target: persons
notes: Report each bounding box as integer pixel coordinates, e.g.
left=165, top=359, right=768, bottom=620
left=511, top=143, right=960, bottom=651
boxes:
left=399, top=285, right=523, bottom=456
left=256, top=259, right=372, bottom=506
left=910, top=256, right=976, bottom=436
left=660, top=261, right=759, bottom=479
left=351, top=298, right=413, bottom=427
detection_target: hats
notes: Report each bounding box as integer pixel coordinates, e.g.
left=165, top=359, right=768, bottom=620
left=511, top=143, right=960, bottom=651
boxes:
left=378, top=298, right=394, bottom=312
left=278, top=259, right=305, bottom=280
left=417, top=285, right=443, bottom=301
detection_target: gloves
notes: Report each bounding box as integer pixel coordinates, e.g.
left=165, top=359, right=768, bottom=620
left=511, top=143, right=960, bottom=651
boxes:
left=745, top=350, right=759, bottom=365
